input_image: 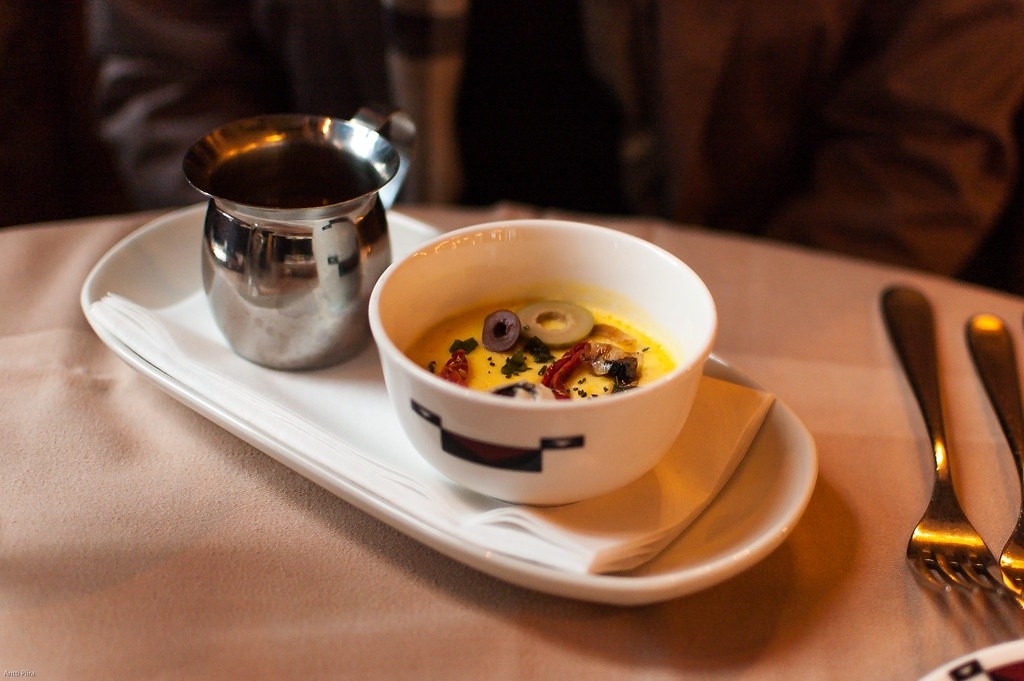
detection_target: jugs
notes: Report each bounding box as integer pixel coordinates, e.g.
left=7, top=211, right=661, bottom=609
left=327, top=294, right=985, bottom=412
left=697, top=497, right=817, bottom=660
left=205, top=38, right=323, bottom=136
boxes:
left=182, top=102, right=420, bottom=371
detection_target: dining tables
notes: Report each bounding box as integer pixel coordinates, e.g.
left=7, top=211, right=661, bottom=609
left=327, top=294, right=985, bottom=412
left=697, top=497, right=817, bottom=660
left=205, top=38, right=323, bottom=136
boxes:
left=0, top=211, right=1024, bottom=681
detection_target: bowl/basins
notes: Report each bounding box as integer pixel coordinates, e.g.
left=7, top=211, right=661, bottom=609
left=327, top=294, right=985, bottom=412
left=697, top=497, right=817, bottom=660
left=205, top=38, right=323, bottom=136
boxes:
left=366, top=218, right=718, bottom=508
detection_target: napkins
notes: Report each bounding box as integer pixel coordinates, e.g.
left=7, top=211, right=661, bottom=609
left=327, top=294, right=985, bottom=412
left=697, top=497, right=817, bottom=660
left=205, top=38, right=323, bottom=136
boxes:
left=91, top=218, right=777, bottom=578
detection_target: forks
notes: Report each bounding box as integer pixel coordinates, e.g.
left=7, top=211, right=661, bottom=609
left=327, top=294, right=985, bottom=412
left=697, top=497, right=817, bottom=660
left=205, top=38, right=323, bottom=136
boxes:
left=880, top=285, right=1020, bottom=600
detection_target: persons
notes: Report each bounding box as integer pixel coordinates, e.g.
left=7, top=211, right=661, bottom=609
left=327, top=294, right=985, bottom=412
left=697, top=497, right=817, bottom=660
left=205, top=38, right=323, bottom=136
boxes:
left=83, top=0, right=1024, bottom=280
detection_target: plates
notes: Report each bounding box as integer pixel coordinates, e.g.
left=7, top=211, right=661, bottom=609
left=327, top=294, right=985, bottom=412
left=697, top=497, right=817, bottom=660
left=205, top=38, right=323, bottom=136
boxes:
left=917, top=637, right=1024, bottom=681
left=79, top=196, right=818, bottom=604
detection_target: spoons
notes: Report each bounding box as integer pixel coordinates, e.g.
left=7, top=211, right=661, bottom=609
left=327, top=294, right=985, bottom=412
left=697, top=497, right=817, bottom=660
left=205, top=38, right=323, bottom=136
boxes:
left=963, top=308, right=1024, bottom=611
